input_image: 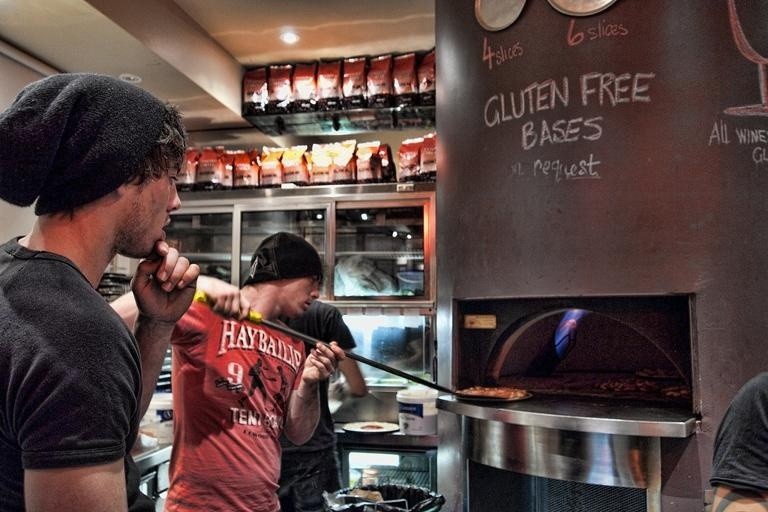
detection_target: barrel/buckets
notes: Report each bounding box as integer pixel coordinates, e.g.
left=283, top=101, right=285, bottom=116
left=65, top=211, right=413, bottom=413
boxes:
left=141, top=394, right=177, bottom=445
left=396, top=388, right=437, bottom=436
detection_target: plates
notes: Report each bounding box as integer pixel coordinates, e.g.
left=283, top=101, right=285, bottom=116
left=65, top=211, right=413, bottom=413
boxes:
left=342, top=421, right=399, bottom=432
left=367, top=385, right=406, bottom=392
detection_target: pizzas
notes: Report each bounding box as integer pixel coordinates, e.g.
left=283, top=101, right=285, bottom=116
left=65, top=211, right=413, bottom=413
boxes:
left=455, top=386, right=528, bottom=398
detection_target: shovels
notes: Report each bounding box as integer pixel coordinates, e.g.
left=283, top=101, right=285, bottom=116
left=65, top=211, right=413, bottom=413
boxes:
left=194, top=291, right=533, bottom=403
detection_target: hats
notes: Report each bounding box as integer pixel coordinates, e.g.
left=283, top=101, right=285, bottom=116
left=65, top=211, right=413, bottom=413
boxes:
left=0, top=72, right=164, bottom=207
left=238, top=228, right=320, bottom=287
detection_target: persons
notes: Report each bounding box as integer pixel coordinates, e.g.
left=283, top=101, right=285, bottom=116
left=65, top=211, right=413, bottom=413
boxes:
left=276, top=297, right=369, bottom=512
left=108, top=229, right=347, bottom=512
left=708, top=371, right=767, bottom=512
left=0, top=71, right=204, bottom=512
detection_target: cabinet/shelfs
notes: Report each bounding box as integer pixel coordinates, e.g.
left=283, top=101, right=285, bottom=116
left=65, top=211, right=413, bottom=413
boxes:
left=128, top=177, right=437, bottom=500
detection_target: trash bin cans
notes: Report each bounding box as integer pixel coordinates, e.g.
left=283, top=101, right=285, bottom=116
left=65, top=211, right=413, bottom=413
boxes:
left=321, top=483, right=446, bottom=512
